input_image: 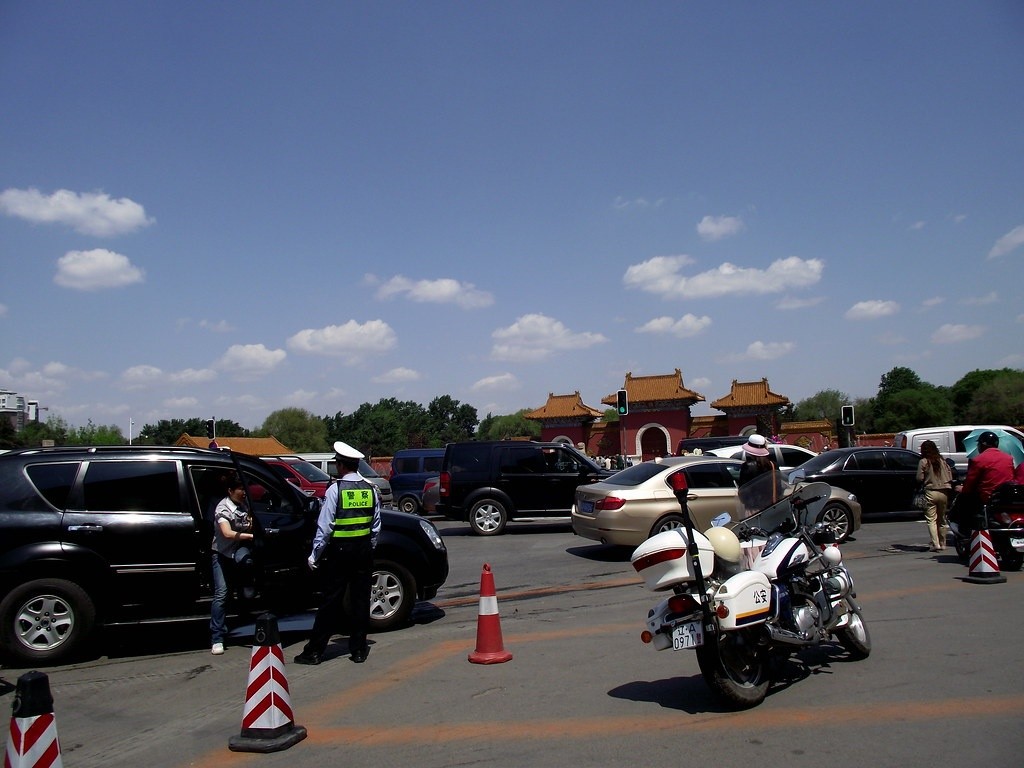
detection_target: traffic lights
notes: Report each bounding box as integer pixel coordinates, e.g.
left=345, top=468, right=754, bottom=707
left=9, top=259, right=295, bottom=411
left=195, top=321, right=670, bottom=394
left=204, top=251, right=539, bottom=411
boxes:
left=616, top=390, right=628, bottom=416
left=841, top=406, right=855, bottom=430
left=206, top=418, right=216, bottom=440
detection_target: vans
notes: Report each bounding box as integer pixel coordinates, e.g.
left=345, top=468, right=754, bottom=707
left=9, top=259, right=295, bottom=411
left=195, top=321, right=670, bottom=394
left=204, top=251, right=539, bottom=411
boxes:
left=894, top=426, right=1024, bottom=464
left=390, top=448, right=492, bottom=517
left=290, top=452, right=394, bottom=512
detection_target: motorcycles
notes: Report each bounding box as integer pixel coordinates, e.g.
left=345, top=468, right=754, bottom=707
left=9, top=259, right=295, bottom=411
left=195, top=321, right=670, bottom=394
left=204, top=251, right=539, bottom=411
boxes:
left=630, top=471, right=871, bottom=707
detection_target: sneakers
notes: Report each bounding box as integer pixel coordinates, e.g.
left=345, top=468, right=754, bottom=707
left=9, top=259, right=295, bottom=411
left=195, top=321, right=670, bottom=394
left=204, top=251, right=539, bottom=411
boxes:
left=211, top=643, right=224, bottom=655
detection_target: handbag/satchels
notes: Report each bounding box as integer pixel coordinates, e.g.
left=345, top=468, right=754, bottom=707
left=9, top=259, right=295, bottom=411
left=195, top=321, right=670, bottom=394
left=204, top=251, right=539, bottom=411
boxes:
left=912, top=487, right=926, bottom=510
left=221, top=533, right=275, bottom=588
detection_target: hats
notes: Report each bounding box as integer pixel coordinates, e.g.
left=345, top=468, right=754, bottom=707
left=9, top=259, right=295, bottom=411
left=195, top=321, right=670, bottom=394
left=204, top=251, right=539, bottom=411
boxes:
left=742, top=434, right=770, bottom=457
left=332, top=440, right=365, bottom=463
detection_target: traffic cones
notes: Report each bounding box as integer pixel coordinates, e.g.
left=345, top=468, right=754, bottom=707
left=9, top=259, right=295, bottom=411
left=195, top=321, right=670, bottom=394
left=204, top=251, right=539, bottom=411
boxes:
left=4, top=665, right=61, bottom=768
left=466, top=562, right=515, bottom=665
left=953, top=516, right=1009, bottom=584
left=228, top=611, right=311, bottom=754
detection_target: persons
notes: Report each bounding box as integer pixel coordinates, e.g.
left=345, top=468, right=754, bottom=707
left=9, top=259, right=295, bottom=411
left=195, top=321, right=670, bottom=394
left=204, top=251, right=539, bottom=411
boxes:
left=951, top=431, right=1018, bottom=538
left=293, top=441, right=383, bottom=666
left=208, top=476, right=262, bottom=655
left=826, top=444, right=832, bottom=451
left=590, top=454, right=633, bottom=471
left=884, top=441, right=890, bottom=446
left=916, top=440, right=952, bottom=551
left=738, top=433, right=783, bottom=569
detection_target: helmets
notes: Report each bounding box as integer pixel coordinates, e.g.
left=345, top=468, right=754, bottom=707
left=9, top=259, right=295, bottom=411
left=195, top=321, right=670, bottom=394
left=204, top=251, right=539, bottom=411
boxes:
left=975, top=431, right=999, bottom=447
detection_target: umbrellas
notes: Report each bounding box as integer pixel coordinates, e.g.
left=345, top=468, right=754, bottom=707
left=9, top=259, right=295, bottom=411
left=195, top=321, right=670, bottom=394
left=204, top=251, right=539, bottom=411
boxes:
left=961, top=428, right=1024, bottom=469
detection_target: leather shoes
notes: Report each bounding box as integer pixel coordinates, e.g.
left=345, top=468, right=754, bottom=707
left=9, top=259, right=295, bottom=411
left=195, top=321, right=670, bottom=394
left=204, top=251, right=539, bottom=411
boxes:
left=352, top=652, right=368, bottom=663
left=294, top=653, right=320, bottom=665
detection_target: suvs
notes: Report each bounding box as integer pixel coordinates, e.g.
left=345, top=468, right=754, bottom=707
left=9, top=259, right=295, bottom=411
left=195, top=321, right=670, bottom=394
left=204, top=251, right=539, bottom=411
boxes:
left=441, top=441, right=620, bottom=537
left=675, top=436, right=774, bottom=457
left=1, top=441, right=448, bottom=664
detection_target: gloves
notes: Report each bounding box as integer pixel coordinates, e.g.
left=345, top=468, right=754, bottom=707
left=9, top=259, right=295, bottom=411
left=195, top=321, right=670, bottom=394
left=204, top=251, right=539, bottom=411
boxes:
left=307, top=555, right=318, bottom=571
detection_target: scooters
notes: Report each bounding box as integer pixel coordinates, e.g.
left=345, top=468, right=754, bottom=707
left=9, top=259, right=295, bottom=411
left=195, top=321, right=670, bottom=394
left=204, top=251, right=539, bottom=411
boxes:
left=945, top=471, right=1024, bottom=571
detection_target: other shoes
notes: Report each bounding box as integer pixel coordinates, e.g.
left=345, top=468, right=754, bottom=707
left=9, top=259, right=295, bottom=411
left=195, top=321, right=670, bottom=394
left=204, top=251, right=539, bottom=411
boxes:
left=929, top=545, right=945, bottom=552
left=949, top=522, right=969, bottom=539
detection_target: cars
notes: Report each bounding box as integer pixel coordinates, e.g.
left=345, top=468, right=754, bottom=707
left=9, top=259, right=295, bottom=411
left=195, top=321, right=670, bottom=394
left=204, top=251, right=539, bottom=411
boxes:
left=214, top=455, right=333, bottom=502
left=419, top=474, right=440, bottom=518
left=572, top=456, right=861, bottom=550
left=786, top=446, right=961, bottom=523
left=703, top=444, right=821, bottom=489
